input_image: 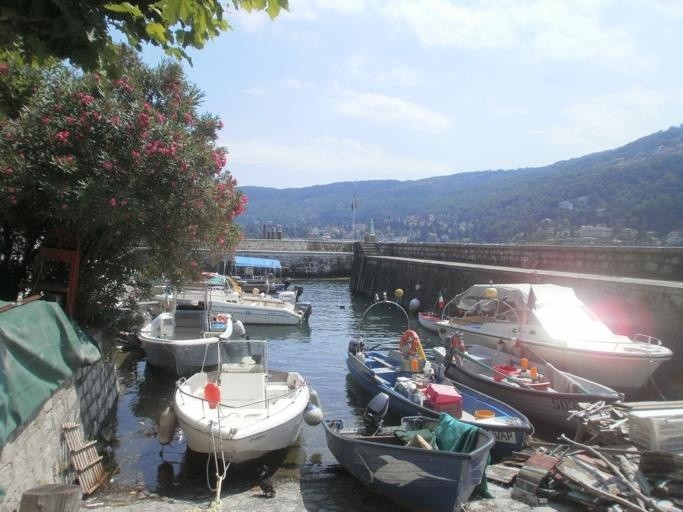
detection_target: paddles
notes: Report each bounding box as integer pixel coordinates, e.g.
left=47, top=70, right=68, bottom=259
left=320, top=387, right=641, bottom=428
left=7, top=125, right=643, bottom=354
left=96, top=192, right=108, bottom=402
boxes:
left=371, top=356, right=412, bottom=377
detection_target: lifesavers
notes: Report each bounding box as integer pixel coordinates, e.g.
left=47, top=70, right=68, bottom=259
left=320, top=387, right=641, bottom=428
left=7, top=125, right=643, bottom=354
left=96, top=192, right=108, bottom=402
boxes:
left=400, top=329, right=421, bottom=357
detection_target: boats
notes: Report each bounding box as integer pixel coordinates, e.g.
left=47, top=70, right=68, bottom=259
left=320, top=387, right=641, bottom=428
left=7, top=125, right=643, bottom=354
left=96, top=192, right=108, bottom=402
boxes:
left=418, top=310, right=442, bottom=332
left=135, top=254, right=311, bottom=377
left=321, top=392, right=497, bottom=512
left=432, top=296, right=626, bottom=431
left=346, top=292, right=535, bottom=459
left=434, top=282, right=675, bottom=390
left=157, top=338, right=324, bottom=466
